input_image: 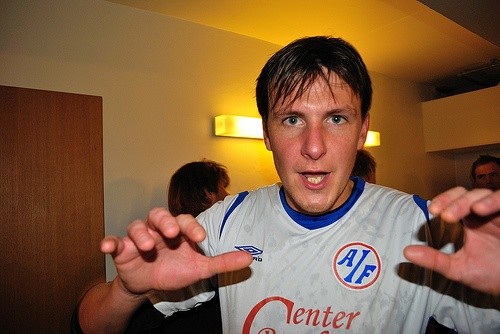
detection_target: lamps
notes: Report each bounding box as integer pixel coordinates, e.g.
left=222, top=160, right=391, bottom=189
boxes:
left=215, top=114, right=380, bottom=149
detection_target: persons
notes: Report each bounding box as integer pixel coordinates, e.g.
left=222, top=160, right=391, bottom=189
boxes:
left=142, top=158, right=236, bottom=334
left=465, top=153, right=500, bottom=194
left=69, top=34, right=500, bottom=334
left=350, top=147, right=378, bottom=185
